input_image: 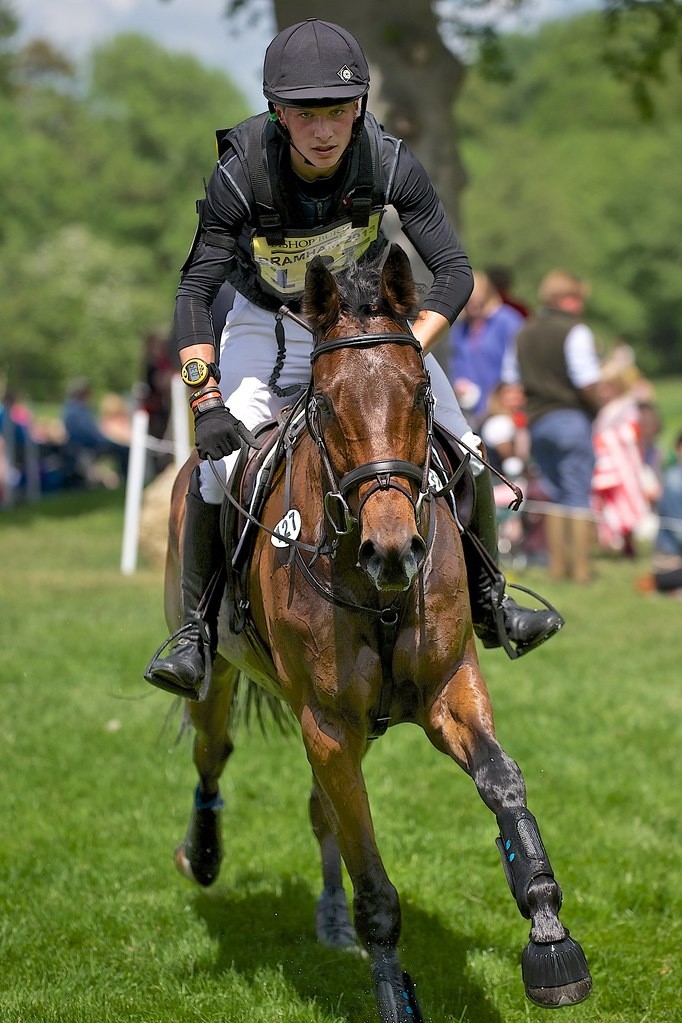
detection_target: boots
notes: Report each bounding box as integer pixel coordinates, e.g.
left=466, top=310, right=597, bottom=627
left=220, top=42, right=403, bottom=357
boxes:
left=152, top=495, right=224, bottom=691
left=457, top=440, right=560, bottom=647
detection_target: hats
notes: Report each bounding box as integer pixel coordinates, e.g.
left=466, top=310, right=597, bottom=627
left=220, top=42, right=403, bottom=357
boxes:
left=537, top=271, right=593, bottom=301
left=262, top=17, right=371, bottom=106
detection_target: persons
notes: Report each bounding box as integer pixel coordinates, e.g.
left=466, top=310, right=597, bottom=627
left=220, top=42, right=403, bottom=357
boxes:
left=445, top=257, right=680, bottom=588
left=133, top=18, right=563, bottom=704
left=0, top=368, right=161, bottom=508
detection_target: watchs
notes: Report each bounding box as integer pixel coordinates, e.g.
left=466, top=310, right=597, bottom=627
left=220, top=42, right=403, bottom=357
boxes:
left=180, top=357, right=223, bottom=386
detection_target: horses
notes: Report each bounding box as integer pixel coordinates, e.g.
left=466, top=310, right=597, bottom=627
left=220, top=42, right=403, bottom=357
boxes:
left=164, top=243, right=594, bottom=1023
